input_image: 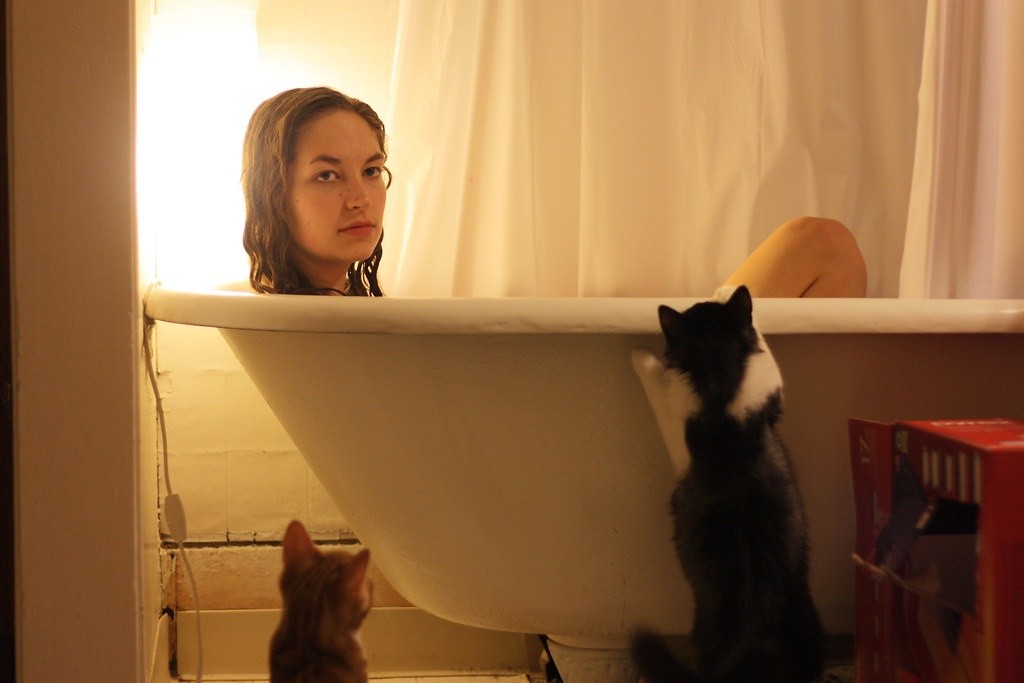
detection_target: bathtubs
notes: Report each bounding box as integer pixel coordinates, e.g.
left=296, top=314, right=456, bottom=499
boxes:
left=139, top=278, right=1023, bottom=638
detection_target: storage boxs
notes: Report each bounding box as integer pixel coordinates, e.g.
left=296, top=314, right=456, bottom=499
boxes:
left=846, top=417, right=1024, bottom=683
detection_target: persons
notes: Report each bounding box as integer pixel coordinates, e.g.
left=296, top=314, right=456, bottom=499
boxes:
left=212, top=86, right=867, bottom=297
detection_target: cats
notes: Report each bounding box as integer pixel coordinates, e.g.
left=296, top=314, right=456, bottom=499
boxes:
left=268, top=519, right=375, bottom=683
left=629, top=284, right=827, bottom=683
